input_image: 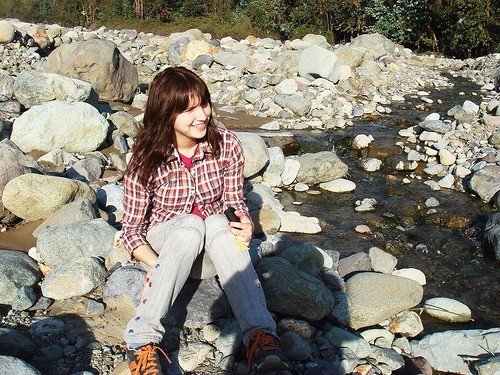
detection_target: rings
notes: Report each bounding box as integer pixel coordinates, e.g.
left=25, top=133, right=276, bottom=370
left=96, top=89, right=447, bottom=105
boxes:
left=244, top=235, right=251, bottom=242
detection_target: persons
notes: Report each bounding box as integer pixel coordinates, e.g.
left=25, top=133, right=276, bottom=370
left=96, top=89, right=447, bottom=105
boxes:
left=117, top=66, right=293, bottom=375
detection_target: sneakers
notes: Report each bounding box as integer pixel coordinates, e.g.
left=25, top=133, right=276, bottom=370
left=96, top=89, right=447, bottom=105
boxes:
left=124, top=345, right=162, bottom=374
left=247, top=331, right=288, bottom=372
